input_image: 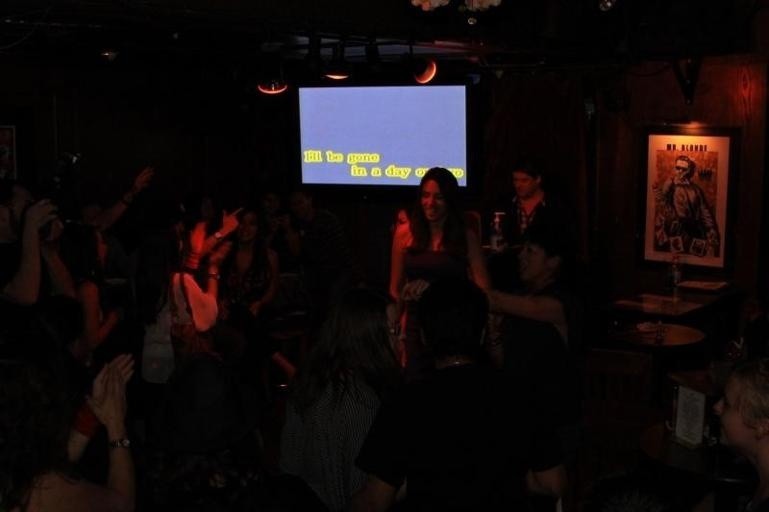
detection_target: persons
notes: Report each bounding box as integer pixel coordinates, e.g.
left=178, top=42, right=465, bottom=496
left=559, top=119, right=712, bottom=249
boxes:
left=1, top=156, right=769, bottom=511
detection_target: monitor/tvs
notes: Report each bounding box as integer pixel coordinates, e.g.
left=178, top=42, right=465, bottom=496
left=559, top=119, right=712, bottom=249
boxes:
left=298, top=85, right=468, bottom=189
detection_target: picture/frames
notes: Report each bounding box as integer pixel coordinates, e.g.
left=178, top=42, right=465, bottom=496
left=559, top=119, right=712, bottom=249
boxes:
left=631, top=120, right=744, bottom=278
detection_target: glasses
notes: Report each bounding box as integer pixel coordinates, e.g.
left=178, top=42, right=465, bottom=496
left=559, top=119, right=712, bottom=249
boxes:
left=676, top=167, right=687, bottom=171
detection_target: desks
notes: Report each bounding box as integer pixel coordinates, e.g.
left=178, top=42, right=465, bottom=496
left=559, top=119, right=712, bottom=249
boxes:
left=609, top=280, right=742, bottom=354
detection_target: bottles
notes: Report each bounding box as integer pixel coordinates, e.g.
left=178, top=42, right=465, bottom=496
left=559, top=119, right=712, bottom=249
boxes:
left=481, top=312, right=504, bottom=367
